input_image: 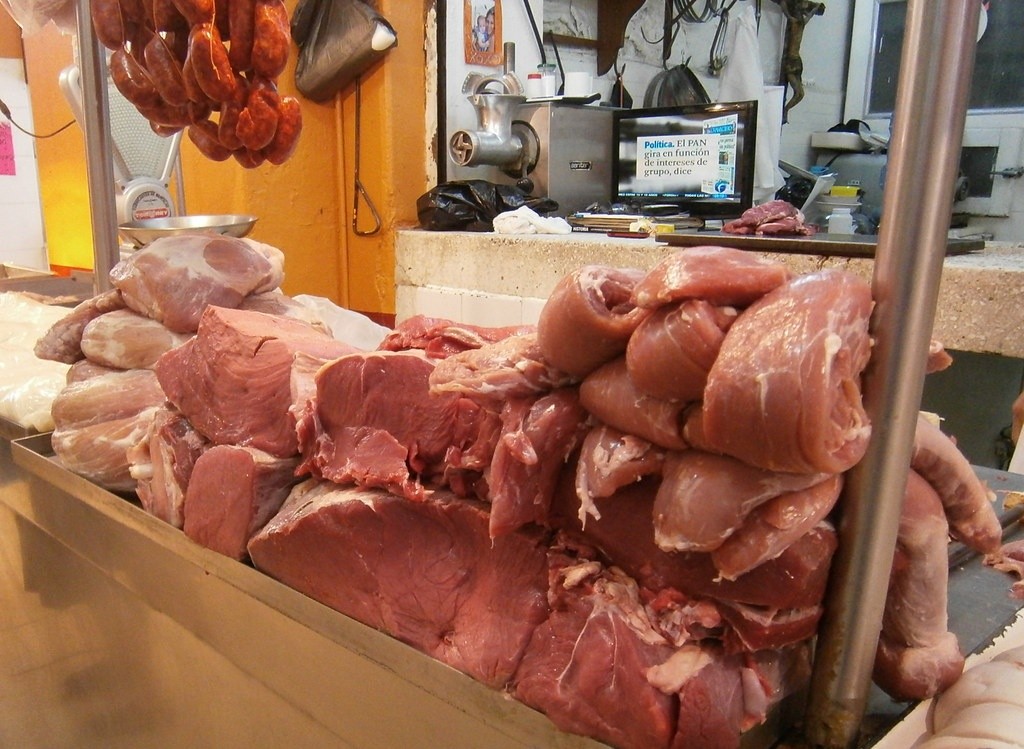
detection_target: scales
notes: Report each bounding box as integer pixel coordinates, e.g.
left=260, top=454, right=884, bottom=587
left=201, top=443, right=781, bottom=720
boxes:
left=59, top=51, right=260, bottom=262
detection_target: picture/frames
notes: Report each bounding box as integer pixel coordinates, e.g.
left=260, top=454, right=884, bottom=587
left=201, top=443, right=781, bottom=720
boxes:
left=463, top=0, right=503, bottom=65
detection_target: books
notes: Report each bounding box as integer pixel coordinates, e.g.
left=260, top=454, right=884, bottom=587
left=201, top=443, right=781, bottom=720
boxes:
left=567, top=213, right=704, bottom=232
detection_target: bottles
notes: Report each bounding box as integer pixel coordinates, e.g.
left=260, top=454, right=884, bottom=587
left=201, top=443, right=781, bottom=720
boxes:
left=827, top=208, right=852, bottom=234
left=527, top=74, right=542, bottom=98
left=537, top=64, right=557, bottom=97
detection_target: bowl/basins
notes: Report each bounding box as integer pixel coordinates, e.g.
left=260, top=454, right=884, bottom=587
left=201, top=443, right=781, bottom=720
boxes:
left=815, top=186, right=862, bottom=213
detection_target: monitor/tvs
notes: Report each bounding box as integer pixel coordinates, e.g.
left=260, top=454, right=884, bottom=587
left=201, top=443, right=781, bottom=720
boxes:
left=610, top=100, right=759, bottom=216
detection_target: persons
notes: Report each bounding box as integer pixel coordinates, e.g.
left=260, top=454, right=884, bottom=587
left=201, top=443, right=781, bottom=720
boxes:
left=1008, top=390, right=1024, bottom=475
left=471, top=5, right=495, bottom=52
